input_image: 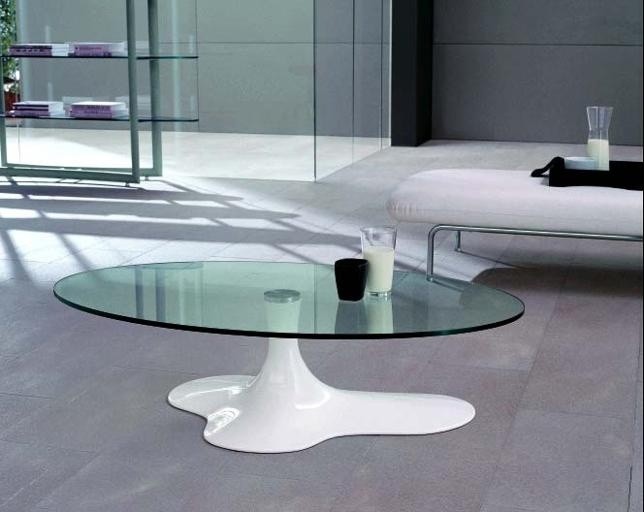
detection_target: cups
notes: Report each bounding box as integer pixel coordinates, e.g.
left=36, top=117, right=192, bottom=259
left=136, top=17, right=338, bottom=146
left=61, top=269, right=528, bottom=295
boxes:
left=334, top=257, right=368, bottom=301
left=586, top=105, right=614, bottom=172
left=360, top=224, right=397, bottom=294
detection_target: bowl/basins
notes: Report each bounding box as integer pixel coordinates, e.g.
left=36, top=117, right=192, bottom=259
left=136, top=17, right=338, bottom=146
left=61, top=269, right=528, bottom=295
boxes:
left=563, top=156, right=596, bottom=171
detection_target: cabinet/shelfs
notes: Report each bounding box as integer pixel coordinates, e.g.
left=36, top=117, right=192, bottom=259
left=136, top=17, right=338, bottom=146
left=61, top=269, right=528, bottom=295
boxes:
left=0, top=47, right=203, bottom=124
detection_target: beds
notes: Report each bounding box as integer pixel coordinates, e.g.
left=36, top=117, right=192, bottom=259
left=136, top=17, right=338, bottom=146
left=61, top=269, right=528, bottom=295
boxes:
left=386, top=155, right=644, bottom=283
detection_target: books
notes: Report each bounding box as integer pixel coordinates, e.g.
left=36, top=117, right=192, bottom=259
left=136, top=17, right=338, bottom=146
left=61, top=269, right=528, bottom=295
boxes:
left=11, top=100, right=65, bottom=115
left=8, top=42, right=125, bottom=56
left=70, top=101, right=126, bottom=118
